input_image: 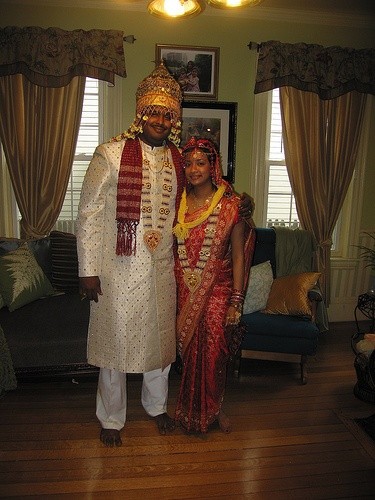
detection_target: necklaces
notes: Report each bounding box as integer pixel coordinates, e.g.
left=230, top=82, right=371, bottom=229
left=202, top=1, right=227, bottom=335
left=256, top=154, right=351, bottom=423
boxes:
left=192, top=188, right=215, bottom=209
left=143, top=151, right=165, bottom=173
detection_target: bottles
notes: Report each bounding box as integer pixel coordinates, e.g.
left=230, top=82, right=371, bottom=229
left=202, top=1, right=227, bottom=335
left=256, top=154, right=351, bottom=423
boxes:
left=293, top=220, right=298, bottom=228
left=280, top=220, right=285, bottom=227
left=274, top=219, right=278, bottom=226
left=268, top=219, right=272, bottom=228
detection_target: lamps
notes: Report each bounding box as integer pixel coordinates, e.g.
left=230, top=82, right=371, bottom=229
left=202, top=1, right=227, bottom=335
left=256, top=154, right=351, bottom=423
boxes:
left=207, top=0, right=261, bottom=9
left=146, top=0, right=202, bottom=19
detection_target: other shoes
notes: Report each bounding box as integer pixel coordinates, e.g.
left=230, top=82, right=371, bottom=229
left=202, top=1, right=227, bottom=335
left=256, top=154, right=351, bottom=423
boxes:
left=156, top=412, right=176, bottom=432
left=218, top=412, right=231, bottom=434
left=100, top=428, right=122, bottom=447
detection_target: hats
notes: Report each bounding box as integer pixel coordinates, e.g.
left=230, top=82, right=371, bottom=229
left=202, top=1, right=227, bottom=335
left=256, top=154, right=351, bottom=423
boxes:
left=109, top=65, right=183, bottom=148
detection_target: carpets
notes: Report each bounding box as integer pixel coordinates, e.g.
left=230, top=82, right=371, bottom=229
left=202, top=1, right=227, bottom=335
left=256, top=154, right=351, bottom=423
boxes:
left=337, top=411, right=375, bottom=461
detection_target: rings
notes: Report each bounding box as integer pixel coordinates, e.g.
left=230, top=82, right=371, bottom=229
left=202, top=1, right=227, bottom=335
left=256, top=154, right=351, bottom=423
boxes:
left=229, top=322, right=232, bottom=325
left=249, top=210, right=251, bottom=213
left=84, top=293, right=86, bottom=295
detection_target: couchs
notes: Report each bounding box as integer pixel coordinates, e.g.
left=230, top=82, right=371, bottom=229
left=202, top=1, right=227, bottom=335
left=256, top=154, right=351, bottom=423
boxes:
left=0, top=228, right=101, bottom=378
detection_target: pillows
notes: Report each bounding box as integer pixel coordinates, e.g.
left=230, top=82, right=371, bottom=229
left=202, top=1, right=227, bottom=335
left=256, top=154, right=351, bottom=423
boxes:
left=242, top=259, right=321, bottom=318
left=0, top=231, right=78, bottom=313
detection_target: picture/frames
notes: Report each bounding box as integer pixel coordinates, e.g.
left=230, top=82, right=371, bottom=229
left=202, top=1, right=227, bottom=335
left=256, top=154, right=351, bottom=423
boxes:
left=180, top=101, right=238, bottom=183
left=155, top=43, right=220, bottom=100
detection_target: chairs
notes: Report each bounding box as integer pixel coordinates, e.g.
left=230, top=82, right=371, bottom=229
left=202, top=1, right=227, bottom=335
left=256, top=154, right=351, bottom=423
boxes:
left=225, top=227, right=322, bottom=385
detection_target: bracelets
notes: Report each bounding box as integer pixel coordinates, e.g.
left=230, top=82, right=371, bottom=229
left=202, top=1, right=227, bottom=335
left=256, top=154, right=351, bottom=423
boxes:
left=230, top=288, right=246, bottom=308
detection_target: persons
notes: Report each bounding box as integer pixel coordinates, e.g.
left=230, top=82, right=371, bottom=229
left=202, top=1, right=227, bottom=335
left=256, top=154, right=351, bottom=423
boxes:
left=74, top=64, right=255, bottom=447
left=184, top=61, right=200, bottom=92
left=171, top=136, right=255, bottom=436
